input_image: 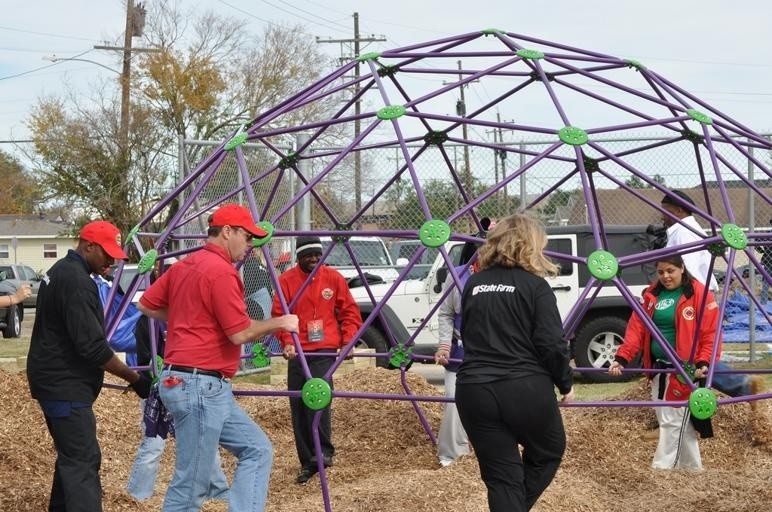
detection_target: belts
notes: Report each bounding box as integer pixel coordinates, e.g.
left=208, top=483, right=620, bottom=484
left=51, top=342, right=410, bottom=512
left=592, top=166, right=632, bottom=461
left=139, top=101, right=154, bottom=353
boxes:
left=162, top=364, right=223, bottom=378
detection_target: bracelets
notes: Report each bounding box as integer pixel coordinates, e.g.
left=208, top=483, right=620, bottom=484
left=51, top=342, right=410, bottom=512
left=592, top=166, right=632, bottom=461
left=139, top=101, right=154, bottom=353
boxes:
left=7, top=295, right=14, bottom=307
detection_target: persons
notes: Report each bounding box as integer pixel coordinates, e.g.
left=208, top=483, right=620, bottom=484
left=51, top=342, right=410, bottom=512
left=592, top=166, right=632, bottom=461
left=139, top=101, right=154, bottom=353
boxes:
left=268, top=232, right=365, bottom=483
left=606, top=252, right=724, bottom=474
left=759, top=218, right=772, bottom=304
left=26, top=220, right=152, bottom=511
left=0, top=281, right=33, bottom=309
left=657, top=188, right=765, bottom=412
left=453, top=213, right=575, bottom=511
left=135, top=202, right=300, bottom=512
left=433, top=245, right=478, bottom=467
left=127, top=257, right=232, bottom=502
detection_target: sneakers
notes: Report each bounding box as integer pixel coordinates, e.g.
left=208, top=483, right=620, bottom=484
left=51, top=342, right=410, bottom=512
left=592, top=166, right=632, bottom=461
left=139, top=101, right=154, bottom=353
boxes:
left=749, top=376, right=762, bottom=412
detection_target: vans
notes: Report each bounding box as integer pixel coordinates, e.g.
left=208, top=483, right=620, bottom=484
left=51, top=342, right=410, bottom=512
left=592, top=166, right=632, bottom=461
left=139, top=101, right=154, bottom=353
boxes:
left=385, top=237, right=440, bottom=281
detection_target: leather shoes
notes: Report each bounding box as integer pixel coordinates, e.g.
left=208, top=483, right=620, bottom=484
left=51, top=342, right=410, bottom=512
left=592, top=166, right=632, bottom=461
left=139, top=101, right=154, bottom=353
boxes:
left=312, top=454, right=333, bottom=467
left=297, top=468, right=317, bottom=481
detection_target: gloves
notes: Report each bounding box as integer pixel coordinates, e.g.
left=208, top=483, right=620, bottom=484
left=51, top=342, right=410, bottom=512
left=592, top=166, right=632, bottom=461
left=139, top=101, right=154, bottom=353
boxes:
left=129, top=372, right=150, bottom=399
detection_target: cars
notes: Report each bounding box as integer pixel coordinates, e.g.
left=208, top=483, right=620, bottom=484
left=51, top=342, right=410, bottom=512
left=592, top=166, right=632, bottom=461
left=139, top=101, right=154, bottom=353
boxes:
left=106, top=263, right=145, bottom=306
left=0, top=271, right=23, bottom=338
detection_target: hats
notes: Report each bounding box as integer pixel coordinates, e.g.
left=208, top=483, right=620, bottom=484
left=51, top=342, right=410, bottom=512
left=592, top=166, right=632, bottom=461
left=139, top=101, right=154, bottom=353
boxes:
left=297, top=235, right=322, bottom=258
left=661, top=190, right=696, bottom=213
left=79, top=221, right=129, bottom=262
left=208, top=205, right=269, bottom=240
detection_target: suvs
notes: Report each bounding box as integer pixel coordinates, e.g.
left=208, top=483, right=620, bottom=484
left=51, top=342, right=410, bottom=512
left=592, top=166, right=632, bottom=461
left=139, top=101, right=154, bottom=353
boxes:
left=0, top=263, right=43, bottom=309
left=345, top=222, right=663, bottom=384
left=279, top=234, right=401, bottom=289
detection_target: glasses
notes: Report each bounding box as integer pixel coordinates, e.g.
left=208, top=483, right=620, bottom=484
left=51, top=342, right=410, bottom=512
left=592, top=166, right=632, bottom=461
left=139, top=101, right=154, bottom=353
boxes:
left=235, top=226, right=254, bottom=242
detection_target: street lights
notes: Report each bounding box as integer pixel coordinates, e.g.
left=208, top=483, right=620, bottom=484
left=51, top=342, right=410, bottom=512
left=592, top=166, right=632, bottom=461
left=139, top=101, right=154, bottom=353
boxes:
left=45, top=54, right=182, bottom=141
